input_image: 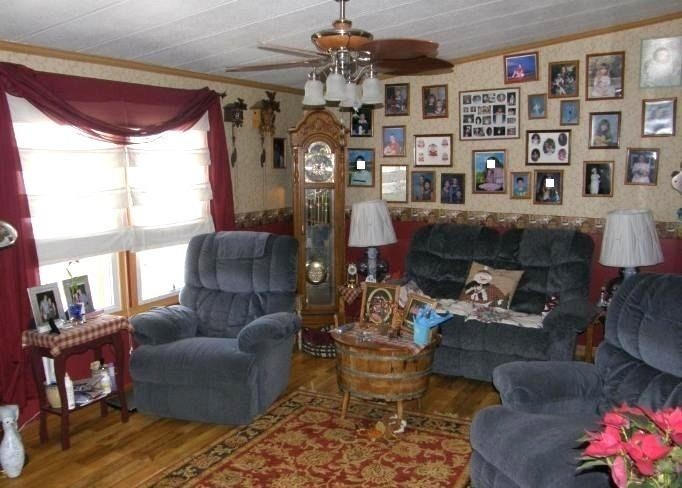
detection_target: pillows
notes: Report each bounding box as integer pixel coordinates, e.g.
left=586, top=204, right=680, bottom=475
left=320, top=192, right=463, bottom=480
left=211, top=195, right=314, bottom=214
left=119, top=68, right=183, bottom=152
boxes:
left=460, top=260, right=525, bottom=312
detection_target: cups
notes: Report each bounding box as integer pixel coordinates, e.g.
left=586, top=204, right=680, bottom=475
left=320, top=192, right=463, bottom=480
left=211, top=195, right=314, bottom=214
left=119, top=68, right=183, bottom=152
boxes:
left=67, top=301, right=87, bottom=325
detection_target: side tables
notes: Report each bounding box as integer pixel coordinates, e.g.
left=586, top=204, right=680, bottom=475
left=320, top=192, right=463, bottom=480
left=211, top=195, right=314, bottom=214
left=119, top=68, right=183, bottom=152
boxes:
left=587, top=312, right=607, bottom=359
left=19, top=315, right=131, bottom=452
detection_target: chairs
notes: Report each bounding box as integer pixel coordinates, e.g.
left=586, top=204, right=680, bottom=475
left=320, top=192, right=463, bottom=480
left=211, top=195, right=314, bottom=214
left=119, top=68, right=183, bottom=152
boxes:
left=129, top=232, right=298, bottom=427
left=472, top=271, right=681, bottom=488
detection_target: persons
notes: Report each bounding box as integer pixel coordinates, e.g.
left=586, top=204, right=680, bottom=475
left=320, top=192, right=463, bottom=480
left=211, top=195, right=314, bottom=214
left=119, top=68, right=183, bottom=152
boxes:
left=589, top=166, right=609, bottom=195
left=463, top=92, right=516, bottom=136
left=390, top=88, right=402, bottom=112
left=73, top=288, right=87, bottom=306
left=537, top=175, right=560, bottom=201
left=355, top=111, right=369, bottom=135
left=594, top=118, right=612, bottom=146
left=40, top=294, right=57, bottom=321
left=514, top=177, right=527, bottom=196
left=553, top=65, right=571, bottom=95
left=351, top=156, right=371, bottom=184
left=512, top=63, right=525, bottom=78
left=442, top=177, right=463, bottom=202
left=478, top=155, right=503, bottom=191
left=416, top=174, right=433, bottom=200
left=428, top=144, right=437, bottom=156
left=631, top=154, right=652, bottom=184
left=425, top=94, right=445, bottom=115
left=591, top=63, right=616, bottom=98
left=532, top=134, right=566, bottom=161
left=644, top=48, right=681, bottom=86
left=384, top=133, right=400, bottom=156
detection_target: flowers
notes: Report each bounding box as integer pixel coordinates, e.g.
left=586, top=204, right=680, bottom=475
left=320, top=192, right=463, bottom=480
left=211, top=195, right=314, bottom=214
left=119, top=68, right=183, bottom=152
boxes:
left=577, top=398, right=682, bottom=488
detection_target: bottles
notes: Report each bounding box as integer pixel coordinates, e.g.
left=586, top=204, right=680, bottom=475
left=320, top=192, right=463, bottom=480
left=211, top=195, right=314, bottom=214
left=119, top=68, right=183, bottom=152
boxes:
left=109, top=362, right=117, bottom=390
left=100, top=367, right=111, bottom=394
left=65, top=372, right=76, bottom=411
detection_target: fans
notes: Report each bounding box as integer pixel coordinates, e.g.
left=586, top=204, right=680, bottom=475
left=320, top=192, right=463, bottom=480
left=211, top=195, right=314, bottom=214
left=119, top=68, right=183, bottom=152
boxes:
left=227, top=0, right=455, bottom=75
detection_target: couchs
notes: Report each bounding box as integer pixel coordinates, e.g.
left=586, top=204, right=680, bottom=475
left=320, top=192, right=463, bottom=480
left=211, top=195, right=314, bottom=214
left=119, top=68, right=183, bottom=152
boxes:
left=392, top=224, right=592, bottom=388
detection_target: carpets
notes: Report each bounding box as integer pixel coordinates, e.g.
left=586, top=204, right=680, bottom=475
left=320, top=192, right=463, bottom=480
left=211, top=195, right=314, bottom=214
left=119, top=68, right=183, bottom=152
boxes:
left=133, top=386, right=474, bottom=487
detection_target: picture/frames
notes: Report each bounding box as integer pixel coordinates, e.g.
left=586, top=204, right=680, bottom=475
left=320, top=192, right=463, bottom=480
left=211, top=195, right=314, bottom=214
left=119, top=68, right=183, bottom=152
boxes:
left=347, top=82, right=465, bottom=205
left=360, top=282, right=399, bottom=332
left=401, top=294, right=437, bottom=334
left=28, top=284, right=62, bottom=336
left=65, top=276, right=94, bottom=322
left=269, top=135, right=288, bottom=170
left=458, top=36, right=681, bottom=203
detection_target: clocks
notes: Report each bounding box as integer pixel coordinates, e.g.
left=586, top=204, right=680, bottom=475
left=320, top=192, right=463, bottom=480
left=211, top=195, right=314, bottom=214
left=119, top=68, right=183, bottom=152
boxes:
left=288, top=109, right=347, bottom=352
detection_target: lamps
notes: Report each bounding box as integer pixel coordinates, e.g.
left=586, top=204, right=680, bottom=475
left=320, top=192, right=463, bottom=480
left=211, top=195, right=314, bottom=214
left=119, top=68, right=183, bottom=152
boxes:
left=597, top=209, right=666, bottom=288
left=346, top=201, right=403, bottom=283
left=303, top=51, right=383, bottom=110
